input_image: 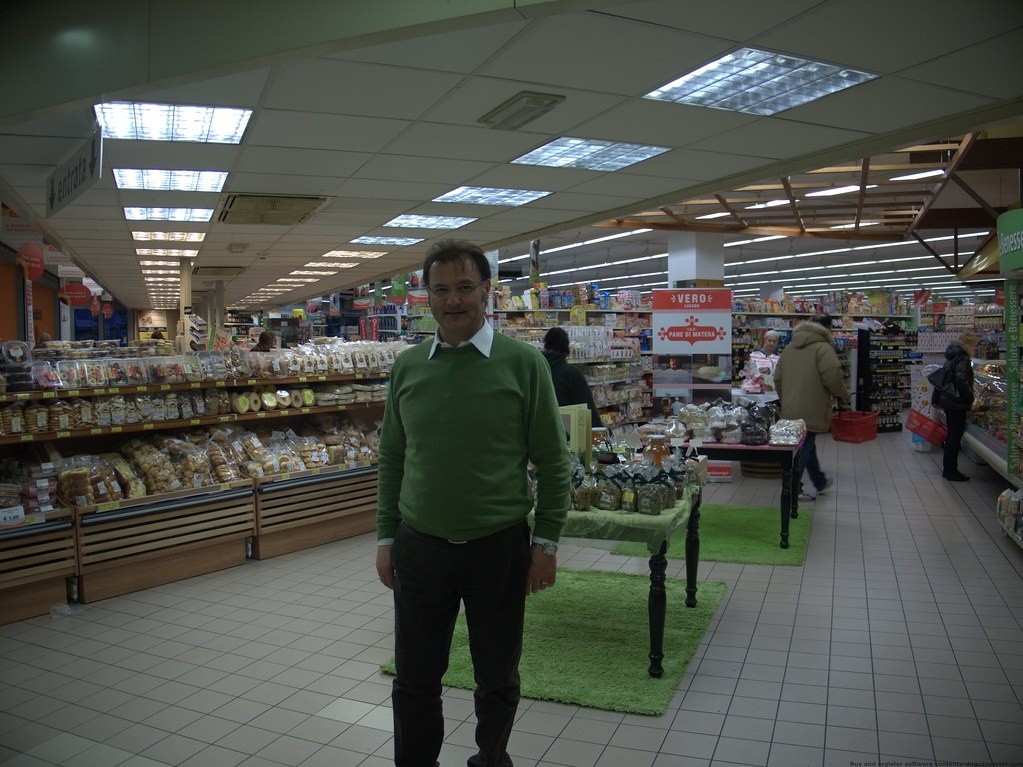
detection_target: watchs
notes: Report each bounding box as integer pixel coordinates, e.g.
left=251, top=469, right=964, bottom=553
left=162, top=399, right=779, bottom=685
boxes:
left=531, top=541, right=558, bottom=557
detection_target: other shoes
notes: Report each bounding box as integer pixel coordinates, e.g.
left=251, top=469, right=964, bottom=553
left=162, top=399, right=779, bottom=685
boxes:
left=942, top=472, right=970, bottom=482
left=797, top=491, right=816, bottom=502
left=816, top=477, right=834, bottom=494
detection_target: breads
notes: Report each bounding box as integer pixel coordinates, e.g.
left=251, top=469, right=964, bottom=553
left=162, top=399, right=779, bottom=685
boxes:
left=31, top=339, right=96, bottom=360
left=96, top=340, right=406, bottom=503
left=0, top=439, right=95, bottom=509
left=528, top=397, right=805, bottom=515
left=582, top=364, right=645, bottom=425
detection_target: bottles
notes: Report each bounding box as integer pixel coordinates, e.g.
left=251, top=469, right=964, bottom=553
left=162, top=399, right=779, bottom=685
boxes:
left=868, top=375, right=911, bottom=428
left=732, top=314, right=752, bottom=380
left=591, top=427, right=670, bottom=466
left=374, top=304, right=397, bottom=343
left=899, top=327, right=918, bottom=346
left=228, top=314, right=254, bottom=335
left=831, top=370, right=852, bottom=414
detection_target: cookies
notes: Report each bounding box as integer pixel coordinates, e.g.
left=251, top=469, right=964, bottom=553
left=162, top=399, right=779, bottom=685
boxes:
left=0, top=400, right=96, bottom=434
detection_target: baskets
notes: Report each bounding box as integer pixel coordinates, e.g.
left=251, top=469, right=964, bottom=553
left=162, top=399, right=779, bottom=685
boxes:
left=831, top=391, right=878, bottom=443
left=905, top=405, right=948, bottom=446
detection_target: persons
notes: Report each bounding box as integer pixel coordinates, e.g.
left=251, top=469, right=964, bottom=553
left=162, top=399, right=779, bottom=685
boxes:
left=774, top=312, right=853, bottom=502
left=753, top=331, right=779, bottom=358
left=250, top=331, right=275, bottom=353
left=33, top=333, right=51, bottom=349
left=540, top=327, right=605, bottom=428
left=665, top=359, right=683, bottom=370
left=376, top=240, right=570, bottom=767
left=151, top=332, right=164, bottom=339
left=933, top=332, right=977, bottom=481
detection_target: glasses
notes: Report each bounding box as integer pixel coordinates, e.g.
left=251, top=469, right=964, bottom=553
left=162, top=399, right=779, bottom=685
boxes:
left=425, top=278, right=491, bottom=296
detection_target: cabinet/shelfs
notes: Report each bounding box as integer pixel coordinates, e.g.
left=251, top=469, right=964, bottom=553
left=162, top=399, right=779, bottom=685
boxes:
left=299, top=311, right=1004, bottom=432
left=0, top=370, right=391, bottom=626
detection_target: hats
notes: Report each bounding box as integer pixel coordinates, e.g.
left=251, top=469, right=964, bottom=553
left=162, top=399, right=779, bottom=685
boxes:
left=544, top=326, right=569, bottom=352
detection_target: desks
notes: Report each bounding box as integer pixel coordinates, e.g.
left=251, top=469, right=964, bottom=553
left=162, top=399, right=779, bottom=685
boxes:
left=671, top=429, right=807, bottom=550
left=526, top=478, right=708, bottom=677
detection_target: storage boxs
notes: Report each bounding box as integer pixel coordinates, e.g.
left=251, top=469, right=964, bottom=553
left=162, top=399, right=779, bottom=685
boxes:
left=831, top=392, right=878, bottom=443
left=904, top=407, right=948, bottom=445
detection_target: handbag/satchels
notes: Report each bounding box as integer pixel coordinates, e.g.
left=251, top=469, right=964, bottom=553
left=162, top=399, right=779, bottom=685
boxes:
left=927, top=354, right=961, bottom=398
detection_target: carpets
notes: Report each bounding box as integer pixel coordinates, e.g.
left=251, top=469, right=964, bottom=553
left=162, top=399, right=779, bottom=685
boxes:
left=610, top=504, right=815, bottom=566
left=380, top=569, right=728, bottom=717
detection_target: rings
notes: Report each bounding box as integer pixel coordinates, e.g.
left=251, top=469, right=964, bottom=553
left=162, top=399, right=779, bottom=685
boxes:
left=542, top=581, right=548, bottom=585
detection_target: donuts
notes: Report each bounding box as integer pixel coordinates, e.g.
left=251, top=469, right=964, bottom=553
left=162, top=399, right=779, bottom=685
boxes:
left=60, top=364, right=106, bottom=387
